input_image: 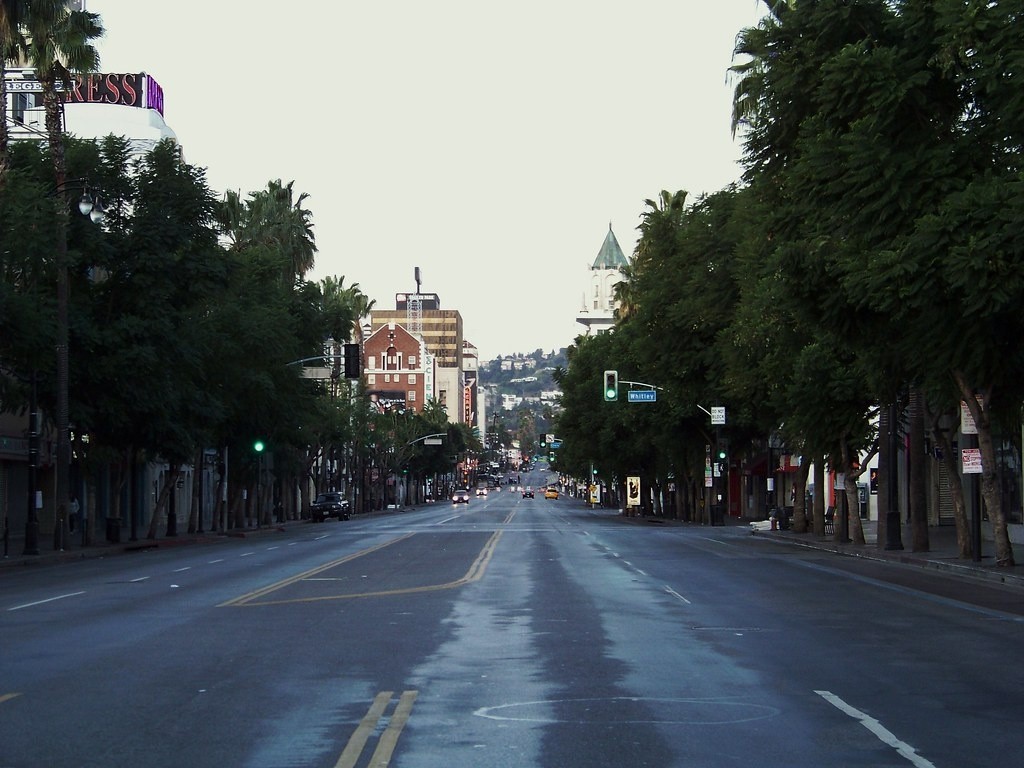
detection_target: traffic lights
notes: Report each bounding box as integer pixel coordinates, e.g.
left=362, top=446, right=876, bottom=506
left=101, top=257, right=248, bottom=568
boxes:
left=604, top=370, right=618, bottom=402
left=540, top=434, right=546, bottom=448
left=593, top=463, right=599, bottom=475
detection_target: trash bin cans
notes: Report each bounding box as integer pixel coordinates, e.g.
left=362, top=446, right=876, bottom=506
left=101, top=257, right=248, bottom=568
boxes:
left=276, top=506, right=286, bottom=523
left=103, top=516, right=123, bottom=543
left=426, top=495, right=431, bottom=502
left=710, top=504, right=726, bottom=527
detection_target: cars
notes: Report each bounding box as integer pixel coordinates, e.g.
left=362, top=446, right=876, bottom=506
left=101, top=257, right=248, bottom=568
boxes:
left=452, top=486, right=558, bottom=504
left=311, top=491, right=351, bottom=523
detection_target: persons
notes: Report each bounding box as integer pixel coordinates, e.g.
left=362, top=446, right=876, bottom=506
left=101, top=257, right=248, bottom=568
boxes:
left=68, top=490, right=79, bottom=536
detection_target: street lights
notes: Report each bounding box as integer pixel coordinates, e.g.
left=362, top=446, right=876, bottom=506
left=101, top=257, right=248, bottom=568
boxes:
left=22, top=177, right=105, bottom=556
left=254, top=438, right=265, bottom=529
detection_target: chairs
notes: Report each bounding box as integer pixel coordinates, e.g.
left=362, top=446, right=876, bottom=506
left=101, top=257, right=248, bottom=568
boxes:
left=824, top=506, right=835, bottom=535
